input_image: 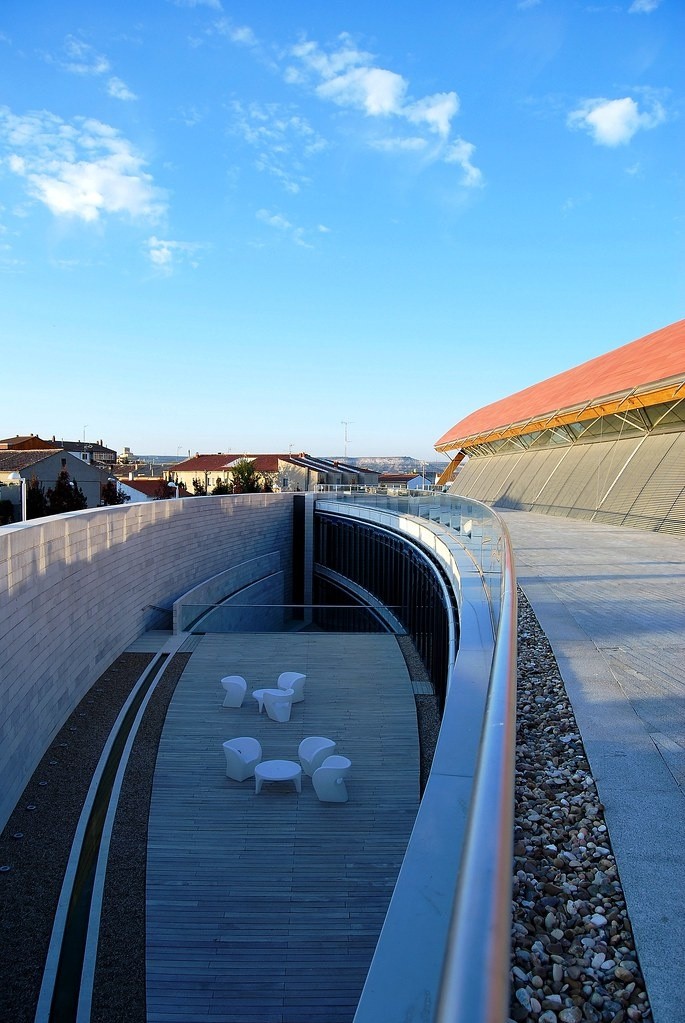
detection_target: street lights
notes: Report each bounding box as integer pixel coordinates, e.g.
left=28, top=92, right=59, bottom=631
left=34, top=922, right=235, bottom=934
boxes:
left=8, top=472, right=27, bottom=522
left=167, top=482, right=179, bottom=498
left=422, top=463, right=429, bottom=490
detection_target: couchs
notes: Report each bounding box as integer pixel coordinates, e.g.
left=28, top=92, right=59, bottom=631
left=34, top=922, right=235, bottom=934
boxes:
left=220, top=676, right=247, bottom=707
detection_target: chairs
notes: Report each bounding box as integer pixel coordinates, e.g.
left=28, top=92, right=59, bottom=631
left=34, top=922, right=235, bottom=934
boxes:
left=223, top=737, right=262, bottom=782
left=263, top=672, right=307, bottom=722
left=298, top=737, right=351, bottom=802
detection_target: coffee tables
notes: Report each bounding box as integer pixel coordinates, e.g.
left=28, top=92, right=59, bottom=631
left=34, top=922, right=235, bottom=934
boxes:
left=255, top=760, right=302, bottom=794
left=252, top=689, right=281, bottom=713
